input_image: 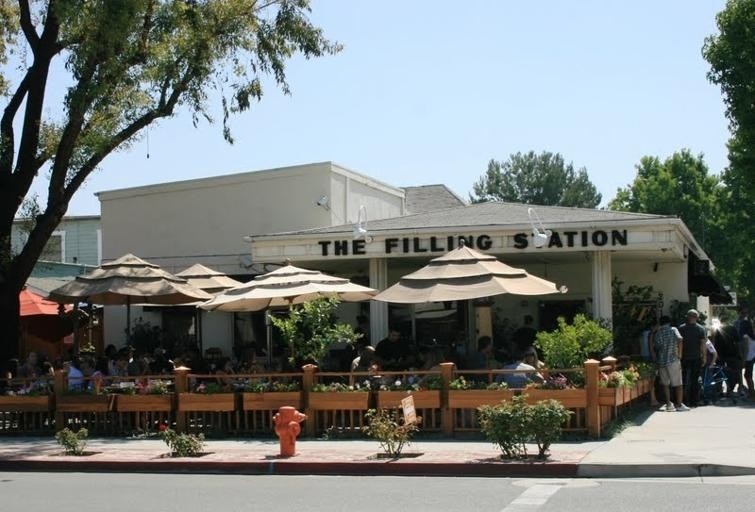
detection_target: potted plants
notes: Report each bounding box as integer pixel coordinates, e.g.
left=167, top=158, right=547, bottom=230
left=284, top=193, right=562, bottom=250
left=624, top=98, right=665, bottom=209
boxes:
left=178, top=384, right=236, bottom=397
left=377, top=373, right=443, bottom=398
left=308, top=381, right=370, bottom=397
left=241, top=381, right=303, bottom=397
left=449, top=371, right=515, bottom=398
left=522, top=371, right=589, bottom=396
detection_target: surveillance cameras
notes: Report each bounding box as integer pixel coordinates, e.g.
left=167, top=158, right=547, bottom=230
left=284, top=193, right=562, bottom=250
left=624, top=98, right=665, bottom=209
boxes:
left=317, top=195, right=328, bottom=206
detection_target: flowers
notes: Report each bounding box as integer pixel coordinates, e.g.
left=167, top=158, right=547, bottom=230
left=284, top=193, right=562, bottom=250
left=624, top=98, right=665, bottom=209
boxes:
left=3, top=371, right=176, bottom=397
left=600, top=360, right=659, bottom=392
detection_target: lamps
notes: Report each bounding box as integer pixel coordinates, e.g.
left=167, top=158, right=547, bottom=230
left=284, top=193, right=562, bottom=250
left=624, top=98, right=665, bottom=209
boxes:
left=527, top=207, right=553, bottom=247
left=355, top=205, right=372, bottom=243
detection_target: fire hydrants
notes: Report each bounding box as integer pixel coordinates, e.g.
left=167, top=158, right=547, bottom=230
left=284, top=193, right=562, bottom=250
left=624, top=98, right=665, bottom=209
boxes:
left=271, top=405, right=306, bottom=457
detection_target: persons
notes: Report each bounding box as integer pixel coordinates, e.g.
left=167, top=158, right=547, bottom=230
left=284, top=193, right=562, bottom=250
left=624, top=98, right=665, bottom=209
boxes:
left=639, top=307, right=754, bottom=411
left=1, top=315, right=548, bottom=429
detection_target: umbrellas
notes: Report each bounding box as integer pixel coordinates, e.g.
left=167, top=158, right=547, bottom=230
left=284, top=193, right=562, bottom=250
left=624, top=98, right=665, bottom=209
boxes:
left=19, top=285, right=73, bottom=316
left=372, top=239, right=568, bottom=351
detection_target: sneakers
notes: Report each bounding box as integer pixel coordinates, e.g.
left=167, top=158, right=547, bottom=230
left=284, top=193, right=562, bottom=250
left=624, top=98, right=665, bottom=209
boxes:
left=658, top=401, right=690, bottom=411
